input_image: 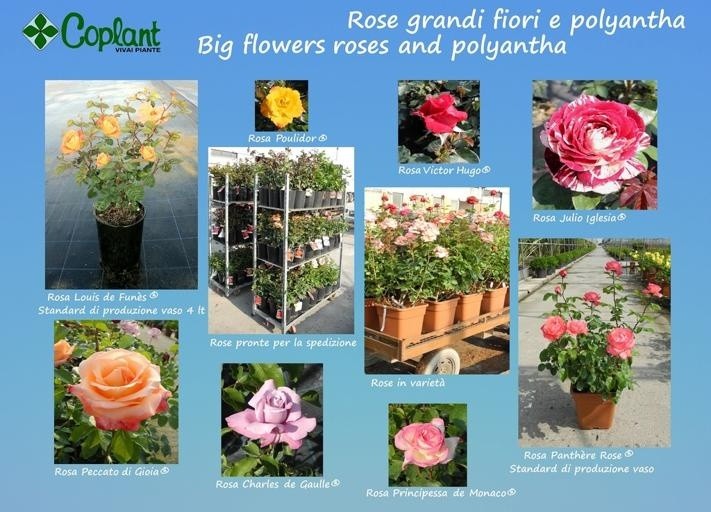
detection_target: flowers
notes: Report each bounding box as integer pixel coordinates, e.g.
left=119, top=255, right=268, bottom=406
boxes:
left=364, top=189, right=510, bottom=308
left=537, top=260, right=665, bottom=405
left=629, top=249, right=671, bottom=271
left=49, top=84, right=199, bottom=218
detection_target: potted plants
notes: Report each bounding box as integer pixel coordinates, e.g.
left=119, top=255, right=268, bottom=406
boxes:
left=530, top=253, right=567, bottom=278
left=208, top=151, right=353, bottom=324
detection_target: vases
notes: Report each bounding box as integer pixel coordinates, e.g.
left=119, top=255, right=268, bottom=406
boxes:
left=364, top=285, right=508, bottom=346
left=93, top=200, right=147, bottom=273
left=645, top=273, right=671, bottom=297
left=569, top=381, right=617, bottom=429
